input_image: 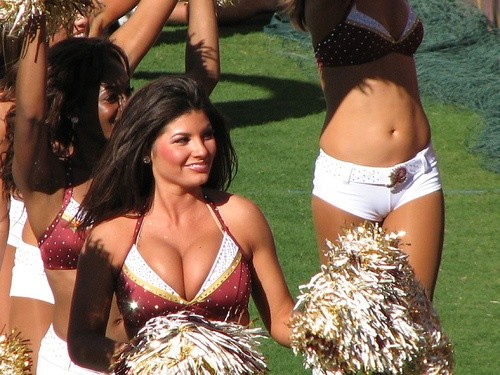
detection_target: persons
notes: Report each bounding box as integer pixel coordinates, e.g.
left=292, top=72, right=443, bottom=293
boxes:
left=65, top=74, right=305, bottom=375
left=0, top=0, right=444, bottom=375
